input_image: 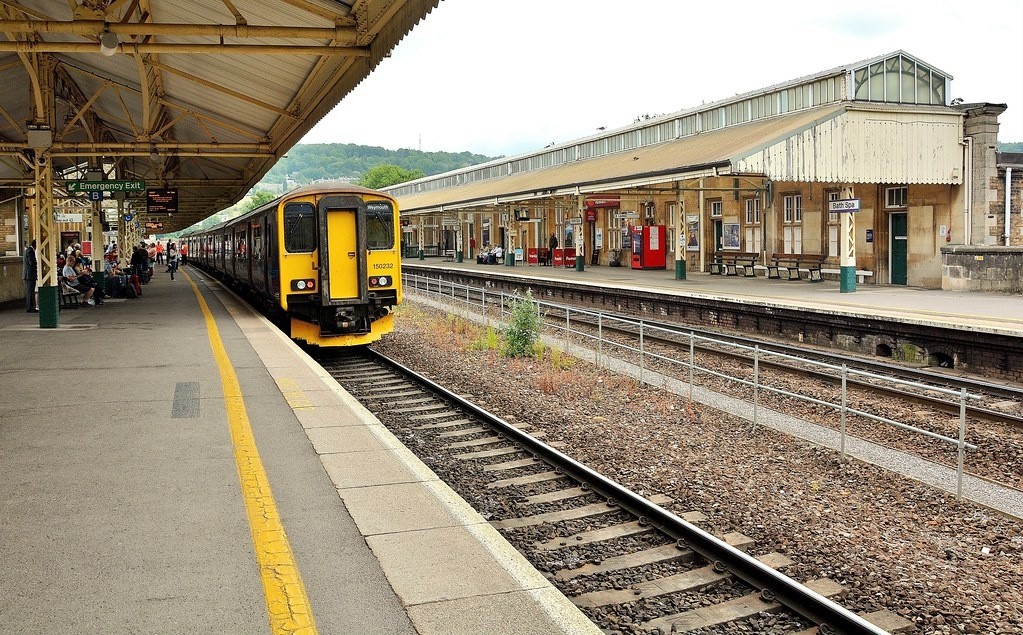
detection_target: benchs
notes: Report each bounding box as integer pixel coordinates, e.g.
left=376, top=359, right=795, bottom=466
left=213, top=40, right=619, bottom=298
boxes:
left=767, top=253, right=828, bottom=283
left=59, top=276, right=81, bottom=309
left=709, top=251, right=759, bottom=277
left=441, top=250, right=456, bottom=262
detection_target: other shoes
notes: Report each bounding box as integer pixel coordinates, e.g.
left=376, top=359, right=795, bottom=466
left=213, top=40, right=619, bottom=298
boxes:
left=82, top=299, right=89, bottom=305
left=27, top=308, right=40, bottom=313
left=96, top=301, right=104, bottom=305
left=171, top=279, right=177, bottom=281
left=102, top=295, right=111, bottom=299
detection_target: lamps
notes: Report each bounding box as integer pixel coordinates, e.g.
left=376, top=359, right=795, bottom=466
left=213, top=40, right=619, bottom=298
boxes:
left=27, top=123, right=52, bottom=131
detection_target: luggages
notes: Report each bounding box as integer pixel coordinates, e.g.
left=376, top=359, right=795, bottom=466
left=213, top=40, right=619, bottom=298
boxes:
left=142, top=269, right=149, bottom=284
left=129, top=274, right=141, bottom=295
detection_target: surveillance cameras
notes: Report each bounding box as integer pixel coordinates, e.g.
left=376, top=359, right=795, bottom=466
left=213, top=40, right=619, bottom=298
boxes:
left=38, top=157, right=46, bottom=165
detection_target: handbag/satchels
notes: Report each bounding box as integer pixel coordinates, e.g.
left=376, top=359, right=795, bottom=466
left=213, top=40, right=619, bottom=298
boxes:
left=126, top=283, right=136, bottom=298
left=77, top=274, right=93, bottom=287
left=150, top=259, right=155, bottom=263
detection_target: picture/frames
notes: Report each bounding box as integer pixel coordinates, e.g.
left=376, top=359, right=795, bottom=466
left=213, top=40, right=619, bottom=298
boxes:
left=723, top=223, right=740, bottom=249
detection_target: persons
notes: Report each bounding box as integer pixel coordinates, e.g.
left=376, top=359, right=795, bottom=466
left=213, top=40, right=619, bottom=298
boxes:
left=22, top=239, right=39, bottom=312
left=480, top=243, right=502, bottom=264
left=549, top=232, right=558, bottom=251
left=57, top=238, right=187, bottom=307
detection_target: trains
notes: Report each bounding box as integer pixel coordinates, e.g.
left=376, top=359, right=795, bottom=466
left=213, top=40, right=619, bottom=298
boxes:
left=179, top=182, right=403, bottom=347
